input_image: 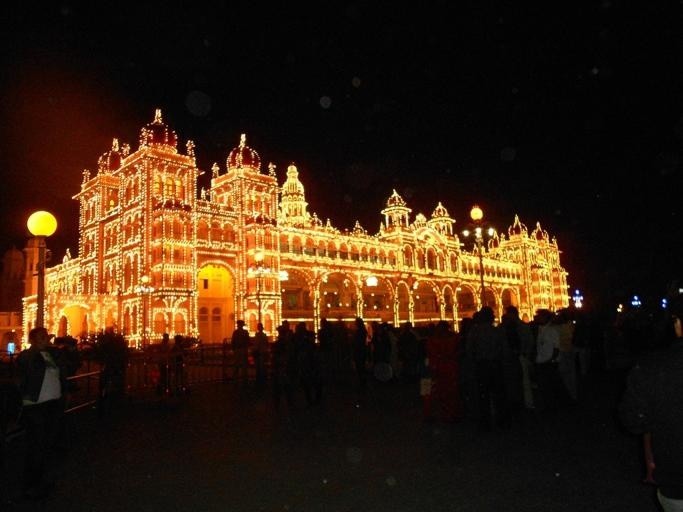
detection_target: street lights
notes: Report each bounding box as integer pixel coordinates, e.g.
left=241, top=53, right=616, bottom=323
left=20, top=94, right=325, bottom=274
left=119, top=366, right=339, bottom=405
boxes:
left=458, top=203, right=496, bottom=307
left=23, top=207, right=61, bottom=328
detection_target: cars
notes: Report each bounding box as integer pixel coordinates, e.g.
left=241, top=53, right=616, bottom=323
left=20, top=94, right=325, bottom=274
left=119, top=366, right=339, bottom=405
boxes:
left=189, top=341, right=252, bottom=369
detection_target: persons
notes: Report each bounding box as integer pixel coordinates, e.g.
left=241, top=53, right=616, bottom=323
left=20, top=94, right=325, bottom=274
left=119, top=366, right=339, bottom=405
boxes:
left=254, top=323, right=269, bottom=385
left=232, top=320, right=252, bottom=384
left=621, top=294, right=683, bottom=510
left=0, top=362, right=23, bottom=512
left=267, top=317, right=366, bottom=413
left=157, top=334, right=171, bottom=399
left=11, top=328, right=71, bottom=502
left=367, top=306, right=670, bottom=434
